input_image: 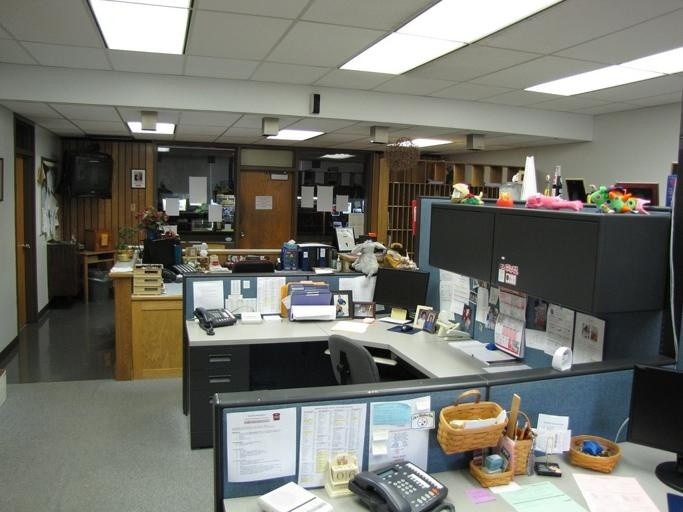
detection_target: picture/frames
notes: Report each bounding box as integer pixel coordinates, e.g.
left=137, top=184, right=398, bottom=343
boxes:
left=328, top=290, right=355, bottom=321
left=613, top=182, right=659, bottom=205
left=413, top=304, right=433, bottom=327
left=352, top=302, right=377, bottom=320
left=423, top=309, right=440, bottom=334
left=565, top=176, right=587, bottom=204
left=131, top=168, right=146, bottom=190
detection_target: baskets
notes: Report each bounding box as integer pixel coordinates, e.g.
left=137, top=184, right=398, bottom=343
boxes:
left=436, top=390, right=508, bottom=455
left=469, top=458, right=512, bottom=488
left=569, top=435, right=621, bottom=474
left=507, top=411, right=532, bottom=475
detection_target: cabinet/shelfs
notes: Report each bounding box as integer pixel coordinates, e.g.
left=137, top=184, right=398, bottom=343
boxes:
left=370, top=154, right=526, bottom=263
left=428, top=199, right=673, bottom=317
left=46, top=239, right=78, bottom=308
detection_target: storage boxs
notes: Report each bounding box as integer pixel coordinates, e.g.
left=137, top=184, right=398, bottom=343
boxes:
left=85, top=227, right=114, bottom=252
left=337, top=252, right=386, bottom=274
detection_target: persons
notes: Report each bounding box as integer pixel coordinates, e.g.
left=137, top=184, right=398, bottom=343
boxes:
left=462, top=303, right=472, bottom=327
left=424, top=314, right=433, bottom=330
left=415, top=310, right=427, bottom=330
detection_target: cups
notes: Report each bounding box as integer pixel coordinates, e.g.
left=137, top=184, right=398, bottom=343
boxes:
left=496, top=184, right=513, bottom=207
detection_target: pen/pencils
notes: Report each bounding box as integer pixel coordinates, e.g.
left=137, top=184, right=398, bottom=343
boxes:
left=514, top=420, right=533, bottom=440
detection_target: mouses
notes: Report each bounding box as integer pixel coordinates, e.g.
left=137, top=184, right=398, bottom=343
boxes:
left=400, top=325, right=413, bottom=331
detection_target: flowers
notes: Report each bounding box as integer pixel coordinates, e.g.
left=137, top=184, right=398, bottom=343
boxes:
left=135, top=206, right=169, bottom=232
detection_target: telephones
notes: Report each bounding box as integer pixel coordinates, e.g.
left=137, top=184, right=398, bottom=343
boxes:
left=162, top=268, right=177, bottom=283
left=192, top=307, right=236, bottom=335
left=347, top=460, right=455, bottom=512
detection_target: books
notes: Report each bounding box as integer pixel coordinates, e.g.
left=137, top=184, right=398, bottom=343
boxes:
left=258, top=480, right=333, bottom=512
left=448, top=338, right=521, bottom=366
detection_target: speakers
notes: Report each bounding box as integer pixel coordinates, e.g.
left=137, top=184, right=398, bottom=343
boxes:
left=310, top=93, right=322, bottom=113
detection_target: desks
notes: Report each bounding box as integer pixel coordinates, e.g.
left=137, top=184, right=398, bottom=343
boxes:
left=180, top=304, right=559, bottom=450
left=109, top=249, right=385, bottom=381
left=222, top=438, right=683, bottom=512
left=73, top=247, right=116, bottom=304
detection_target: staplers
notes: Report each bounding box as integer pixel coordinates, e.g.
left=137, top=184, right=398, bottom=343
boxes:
left=444, top=328, right=473, bottom=342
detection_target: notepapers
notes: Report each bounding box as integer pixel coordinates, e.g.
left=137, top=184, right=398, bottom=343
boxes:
left=464, top=487, right=496, bottom=505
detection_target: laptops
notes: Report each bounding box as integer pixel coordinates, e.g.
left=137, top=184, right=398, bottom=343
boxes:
left=564, top=178, right=596, bottom=208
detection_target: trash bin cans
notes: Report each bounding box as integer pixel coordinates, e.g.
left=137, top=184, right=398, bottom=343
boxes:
left=89, top=270, right=113, bottom=303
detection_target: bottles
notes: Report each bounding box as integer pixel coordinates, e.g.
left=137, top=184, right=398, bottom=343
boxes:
left=551, top=166, right=563, bottom=198
left=543, top=175, right=551, bottom=197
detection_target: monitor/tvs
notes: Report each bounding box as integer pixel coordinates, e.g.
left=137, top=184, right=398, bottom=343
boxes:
left=372, top=267, right=431, bottom=325
left=626, top=363, right=683, bottom=492
left=62, top=153, right=114, bottom=199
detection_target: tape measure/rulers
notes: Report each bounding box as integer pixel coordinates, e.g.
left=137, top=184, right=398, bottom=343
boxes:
left=506, top=393, right=521, bottom=439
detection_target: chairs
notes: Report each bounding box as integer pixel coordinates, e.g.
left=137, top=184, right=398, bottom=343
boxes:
left=231, top=260, right=274, bottom=273
left=328, top=335, right=380, bottom=386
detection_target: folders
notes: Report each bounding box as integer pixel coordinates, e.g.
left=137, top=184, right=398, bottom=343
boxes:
left=288, top=283, right=332, bottom=322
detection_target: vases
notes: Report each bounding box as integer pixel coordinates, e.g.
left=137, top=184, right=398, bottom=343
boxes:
left=147, top=231, right=156, bottom=239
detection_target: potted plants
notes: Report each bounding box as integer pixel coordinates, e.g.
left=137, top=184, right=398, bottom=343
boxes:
left=112, top=226, right=137, bottom=262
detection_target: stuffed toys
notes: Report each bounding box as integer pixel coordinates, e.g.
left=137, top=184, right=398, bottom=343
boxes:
left=587, top=186, right=651, bottom=215
left=383, top=242, right=419, bottom=271
left=523, top=192, right=583, bottom=211
left=350, top=239, right=387, bottom=280
left=451, top=183, right=484, bottom=205
left=496, top=192, right=513, bottom=207
